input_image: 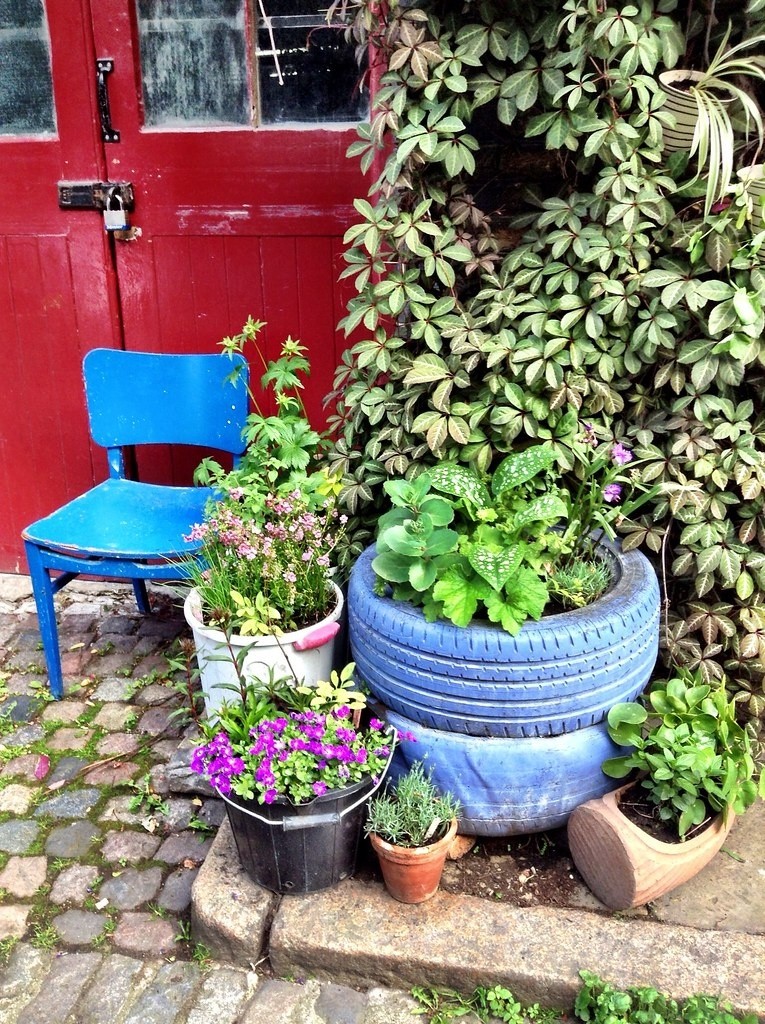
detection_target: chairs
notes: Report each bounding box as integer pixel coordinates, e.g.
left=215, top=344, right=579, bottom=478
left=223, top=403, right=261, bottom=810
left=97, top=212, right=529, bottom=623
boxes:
left=20, top=347, right=250, bottom=692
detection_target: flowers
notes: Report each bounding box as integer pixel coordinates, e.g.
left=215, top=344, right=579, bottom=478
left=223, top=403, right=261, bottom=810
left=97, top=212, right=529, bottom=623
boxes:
left=346, top=426, right=642, bottom=631
left=153, top=482, right=359, bottom=639
left=166, top=605, right=417, bottom=806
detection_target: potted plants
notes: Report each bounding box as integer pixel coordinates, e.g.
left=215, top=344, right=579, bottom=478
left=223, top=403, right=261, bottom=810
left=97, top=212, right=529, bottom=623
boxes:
left=654, top=19, right=765, bottom=223
left=566, top=662, right=763, bottom=910
left=363, top=752, right=465, bottom=904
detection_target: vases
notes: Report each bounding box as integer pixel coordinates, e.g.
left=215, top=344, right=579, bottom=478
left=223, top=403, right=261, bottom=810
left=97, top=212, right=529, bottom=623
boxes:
left=182, top=582, right=342, bottom=728
left=212, top=704, right=398, bottom=892
left=350, top=543, right=665, bottom=836
left=736, top=163, right=765, bottom=264
